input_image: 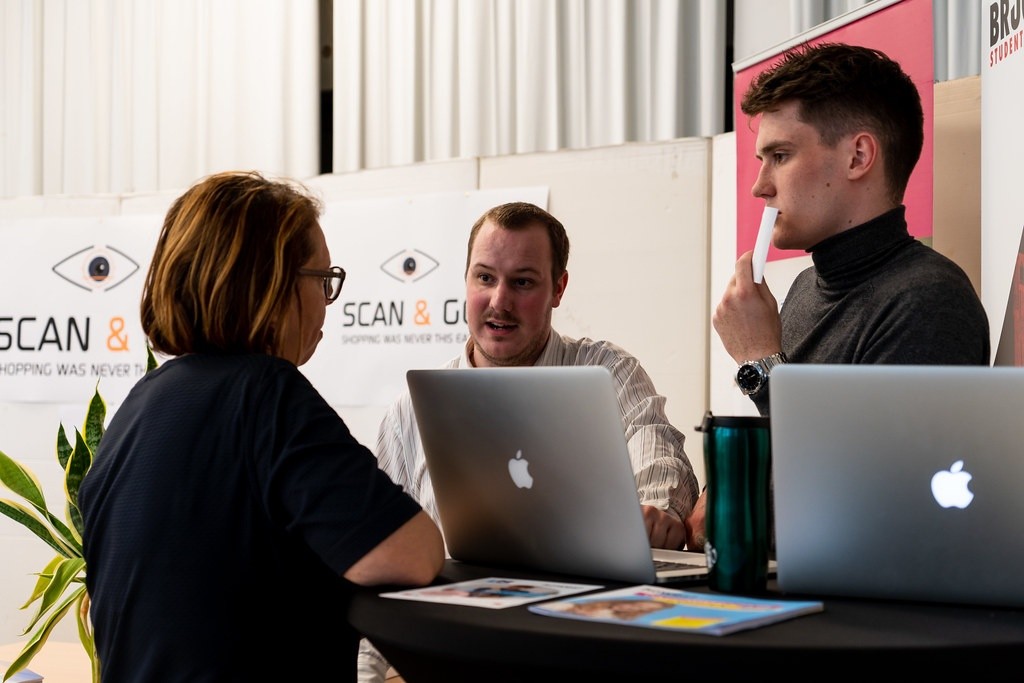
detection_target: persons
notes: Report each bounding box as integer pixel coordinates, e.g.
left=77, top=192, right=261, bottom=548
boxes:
left=685, top=43, right=992, bottom=559
left=357, top=202, right=700, bottom=683
left=75, top=173, right=444, bottom=683
left=552, top=598, right=675, bottom=623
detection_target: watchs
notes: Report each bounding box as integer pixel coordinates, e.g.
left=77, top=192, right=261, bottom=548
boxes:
left=735, top=353, right=789, bottom=395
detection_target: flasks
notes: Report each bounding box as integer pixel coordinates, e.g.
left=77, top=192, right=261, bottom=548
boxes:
left=700, top=414, right=770, bottom=596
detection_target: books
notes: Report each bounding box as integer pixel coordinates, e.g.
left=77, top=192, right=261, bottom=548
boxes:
left=528, top=584, right=824, bottom=637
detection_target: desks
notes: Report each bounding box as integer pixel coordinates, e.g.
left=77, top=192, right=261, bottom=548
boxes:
left=340, top=557, right=1024, bottom=682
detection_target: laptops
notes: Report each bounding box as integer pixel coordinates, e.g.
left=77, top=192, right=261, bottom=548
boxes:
left=768, top=361, right=1023, bottom=613
left=406, top=365, right=778, bottom=590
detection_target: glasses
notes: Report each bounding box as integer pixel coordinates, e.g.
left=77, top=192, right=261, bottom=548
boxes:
left=296, top=266, right=348, bottom=301
